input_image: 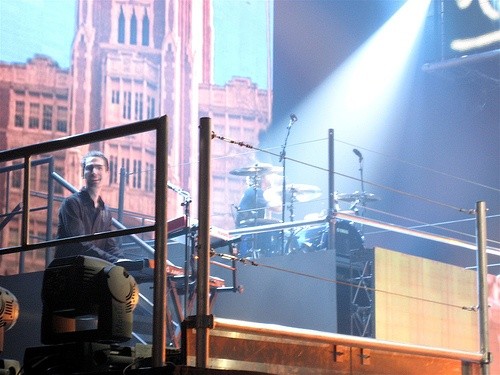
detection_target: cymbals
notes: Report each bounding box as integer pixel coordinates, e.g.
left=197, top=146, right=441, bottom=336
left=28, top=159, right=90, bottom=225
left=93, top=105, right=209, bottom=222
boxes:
left=229, top=164, right=284, bottom=176
left=263, top=185, right=322, bottom=204
left=336, top=193, right=380, bottom=202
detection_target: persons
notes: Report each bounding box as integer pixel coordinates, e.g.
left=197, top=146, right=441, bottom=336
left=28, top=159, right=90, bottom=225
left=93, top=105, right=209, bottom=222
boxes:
left=54, top=152, right=132, bottom=354
left=235, top=163, right=287, bottom=254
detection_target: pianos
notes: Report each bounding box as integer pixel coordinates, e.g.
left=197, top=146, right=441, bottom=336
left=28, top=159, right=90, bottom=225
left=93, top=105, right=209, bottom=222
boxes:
left=166, top=215, right=231, bottom=249
left=113, top=257, right=225, bottom=289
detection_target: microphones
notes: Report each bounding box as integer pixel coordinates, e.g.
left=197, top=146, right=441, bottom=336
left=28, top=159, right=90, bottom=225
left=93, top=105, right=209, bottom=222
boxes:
left=167, top=183, right=178, bottom=192
left=289, top=114, right=297, bottom=122
left=353, top=149, right=363, bottom=157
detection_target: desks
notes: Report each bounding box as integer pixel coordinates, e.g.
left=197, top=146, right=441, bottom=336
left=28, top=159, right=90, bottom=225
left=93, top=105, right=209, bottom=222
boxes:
left=113, top=260, right=223, bottom=349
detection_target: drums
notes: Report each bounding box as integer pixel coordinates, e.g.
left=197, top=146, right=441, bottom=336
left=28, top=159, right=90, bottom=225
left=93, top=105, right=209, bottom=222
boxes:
left=239, top=218, right=281, bottom=256
left=301, top=220, right=365, bottom=252
